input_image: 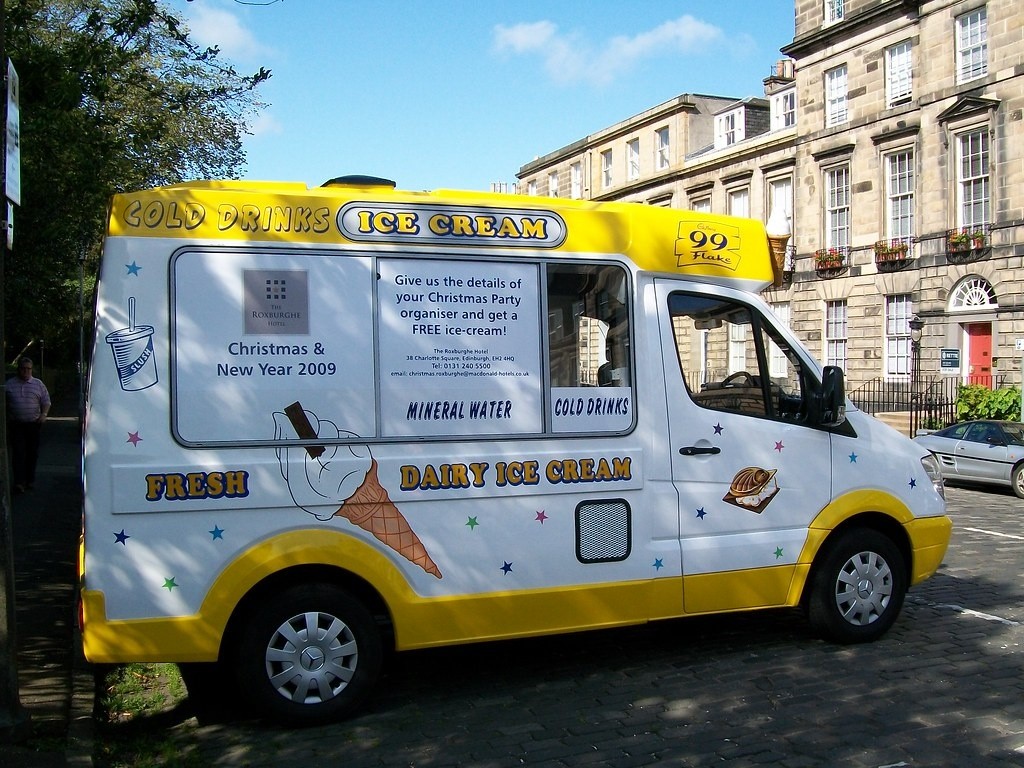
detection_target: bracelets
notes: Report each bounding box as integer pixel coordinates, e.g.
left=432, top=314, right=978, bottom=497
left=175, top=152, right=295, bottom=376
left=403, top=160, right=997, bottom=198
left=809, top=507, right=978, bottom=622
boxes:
left=42, top=413, right=48, bottom=416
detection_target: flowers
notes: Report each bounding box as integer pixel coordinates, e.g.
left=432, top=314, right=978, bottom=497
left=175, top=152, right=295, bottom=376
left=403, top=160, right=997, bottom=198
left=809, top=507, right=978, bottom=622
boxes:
left=824, top=247, right=835, bottom=261
left=897, top=244, right=909, bottom=252
left=959, top=227, right=971, bottom=242
left=948, top=234, right=958, bottom=244
left=830, top=252, right=845, bottom=261
left=871, top=242, right=887, bottom=253
left=815, top=250, right=825, bottom=261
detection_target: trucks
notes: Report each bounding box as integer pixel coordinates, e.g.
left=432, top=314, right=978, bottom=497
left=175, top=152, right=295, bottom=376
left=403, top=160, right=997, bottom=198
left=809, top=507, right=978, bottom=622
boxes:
left=79, top=175, right=954, bottom=727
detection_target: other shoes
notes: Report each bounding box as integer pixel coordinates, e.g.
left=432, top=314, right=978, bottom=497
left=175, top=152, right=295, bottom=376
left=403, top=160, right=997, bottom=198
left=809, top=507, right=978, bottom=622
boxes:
left=27, top=481, right=35, bottom=488
left=15, top=485, right=24, bottom=493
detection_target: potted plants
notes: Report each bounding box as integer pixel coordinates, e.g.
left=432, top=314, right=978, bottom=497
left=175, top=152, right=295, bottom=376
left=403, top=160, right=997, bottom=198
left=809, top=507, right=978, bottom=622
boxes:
left=888, top=248, right=897, bottom=260
left=972, top=231, right=986, bottom=248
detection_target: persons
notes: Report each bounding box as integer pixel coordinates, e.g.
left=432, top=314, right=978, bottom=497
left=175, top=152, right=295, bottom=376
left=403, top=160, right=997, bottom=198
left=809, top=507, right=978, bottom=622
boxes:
left=2, top=358, right=50, bottom=491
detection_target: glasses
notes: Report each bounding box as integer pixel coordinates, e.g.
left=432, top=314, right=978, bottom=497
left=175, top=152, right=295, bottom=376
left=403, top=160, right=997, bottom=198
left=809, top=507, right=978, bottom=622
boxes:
left=21, top=367, right=32, bottom=370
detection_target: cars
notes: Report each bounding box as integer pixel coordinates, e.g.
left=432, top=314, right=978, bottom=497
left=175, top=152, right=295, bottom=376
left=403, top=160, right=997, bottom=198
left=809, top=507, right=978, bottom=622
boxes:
left=912, top=418, right=1024, bottom=499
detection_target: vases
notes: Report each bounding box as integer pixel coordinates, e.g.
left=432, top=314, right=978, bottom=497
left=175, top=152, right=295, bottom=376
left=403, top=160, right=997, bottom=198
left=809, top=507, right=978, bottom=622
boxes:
left=898, top=252, right=906, bottom=259
left=826, top=260, right=832, bottom=268
left=960, top=241, right=969, bottom=250
left=950, top=243, right=957, bottom=251
left=877, top=253, right=887, bottom=262
left=832, top=260, right=842, bottom=267
left=819, top=261, right=825, bottom=268
